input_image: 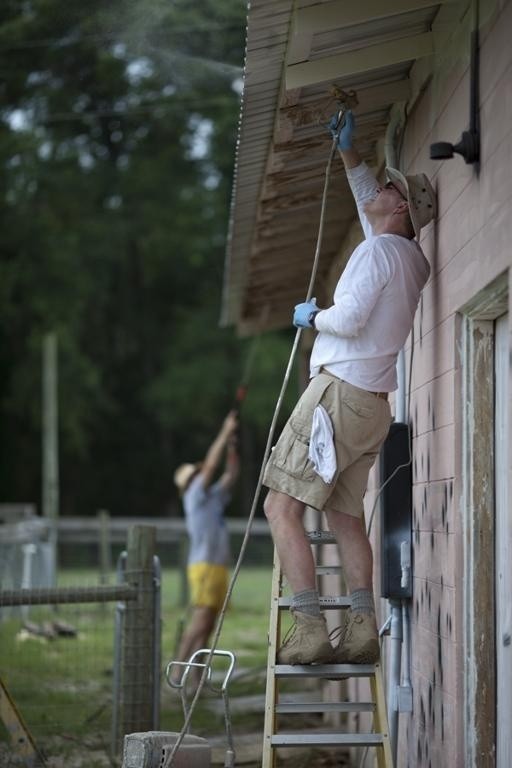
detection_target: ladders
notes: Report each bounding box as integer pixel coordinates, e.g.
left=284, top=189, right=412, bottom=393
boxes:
left=262, top=447, right=389, bottom=766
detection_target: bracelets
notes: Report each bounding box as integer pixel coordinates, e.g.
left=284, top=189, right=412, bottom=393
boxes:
left=307, top=310, right=321, bottom=329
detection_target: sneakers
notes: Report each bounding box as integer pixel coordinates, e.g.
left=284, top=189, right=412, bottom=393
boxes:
left=277, top=610, right=333, bottom=665
left=328, top=609, right=379, bottom=664
left=192, top=684, right=223, bottom=698
left=165, top=681, right=194, bottom=700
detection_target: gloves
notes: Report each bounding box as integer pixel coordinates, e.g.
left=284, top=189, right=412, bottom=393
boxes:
left=292, top=297, right=321, bottom=329
left=328, top=109, right=355, bottom=151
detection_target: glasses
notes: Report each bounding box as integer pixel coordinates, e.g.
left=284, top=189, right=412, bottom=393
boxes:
left=385, top=182, right=407, bottom=201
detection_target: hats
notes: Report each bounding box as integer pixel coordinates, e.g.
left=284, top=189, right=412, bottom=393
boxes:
left=173, top=462, right=203, bottom=499
left=384, top=166, right=437, bottom=243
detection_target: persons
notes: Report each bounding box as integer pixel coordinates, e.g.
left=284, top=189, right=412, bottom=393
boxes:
left=257, top=105, right=441, bottom=680
left=161, top=410, right=242, bottom=698
